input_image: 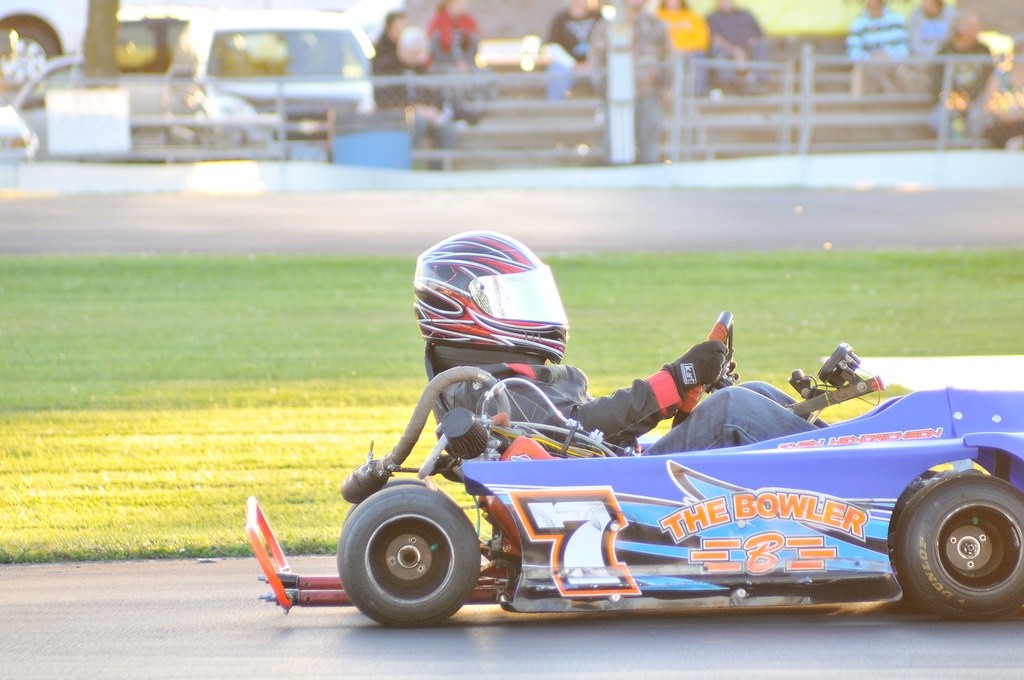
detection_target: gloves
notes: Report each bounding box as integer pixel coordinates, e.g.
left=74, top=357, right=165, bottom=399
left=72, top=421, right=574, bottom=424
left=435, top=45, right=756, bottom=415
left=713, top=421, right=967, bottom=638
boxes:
left=664, top=340, right=739, bottom=401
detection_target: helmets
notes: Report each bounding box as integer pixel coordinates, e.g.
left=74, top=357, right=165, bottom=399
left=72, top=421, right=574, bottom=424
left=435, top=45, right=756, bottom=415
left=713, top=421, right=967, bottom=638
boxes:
left=413, top=230, right=570, bottom=384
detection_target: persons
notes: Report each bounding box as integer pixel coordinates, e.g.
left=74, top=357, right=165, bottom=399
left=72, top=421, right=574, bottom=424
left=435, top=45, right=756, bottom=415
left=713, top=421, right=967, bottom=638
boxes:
left=543, top=0, right=601, bottom=105
left=842, top=0, right=907, bottom=99
left=656, top=0, right=711, bottom=97
left=420, top=0, right=480, bottom=66
left=925, top=13, right=995, bottom=140
left=413, top=231, right=827, bottom=457
left=705, top=0, right=770, bottom=91
left=901, top=0, right=956, bottom=92
left=372, top=14, right=457, bottom=169
left=589, top=0, right=677, bottom=163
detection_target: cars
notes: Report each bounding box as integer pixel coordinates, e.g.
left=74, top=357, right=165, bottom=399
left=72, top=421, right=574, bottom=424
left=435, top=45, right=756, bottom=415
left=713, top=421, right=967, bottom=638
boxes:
left=162, top=12, right=375, bottom=139
left=0, top=2, right=89, bottom=109
